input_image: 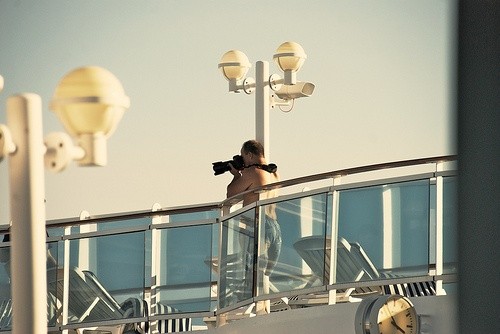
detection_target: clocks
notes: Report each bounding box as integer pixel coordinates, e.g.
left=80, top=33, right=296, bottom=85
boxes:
left=354, top=295, right=420, bottom=334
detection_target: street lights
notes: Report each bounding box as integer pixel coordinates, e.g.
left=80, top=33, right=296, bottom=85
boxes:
left=0, top=63, right=133, bottom=334
left=217, top=40, right=308, bottom=162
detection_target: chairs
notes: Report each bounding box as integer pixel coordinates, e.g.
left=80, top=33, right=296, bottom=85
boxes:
left=0, top=235, right=442, bottom=334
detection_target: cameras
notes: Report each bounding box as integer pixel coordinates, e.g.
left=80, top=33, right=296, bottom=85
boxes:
left=212, top=155, right=245, bottom=175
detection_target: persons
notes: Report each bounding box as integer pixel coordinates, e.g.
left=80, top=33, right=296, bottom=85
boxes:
left=227, top=140, right=281, bottom=314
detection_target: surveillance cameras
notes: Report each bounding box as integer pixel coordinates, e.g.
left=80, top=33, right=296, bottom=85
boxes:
left=276, top=80, right=316, bottom=99
